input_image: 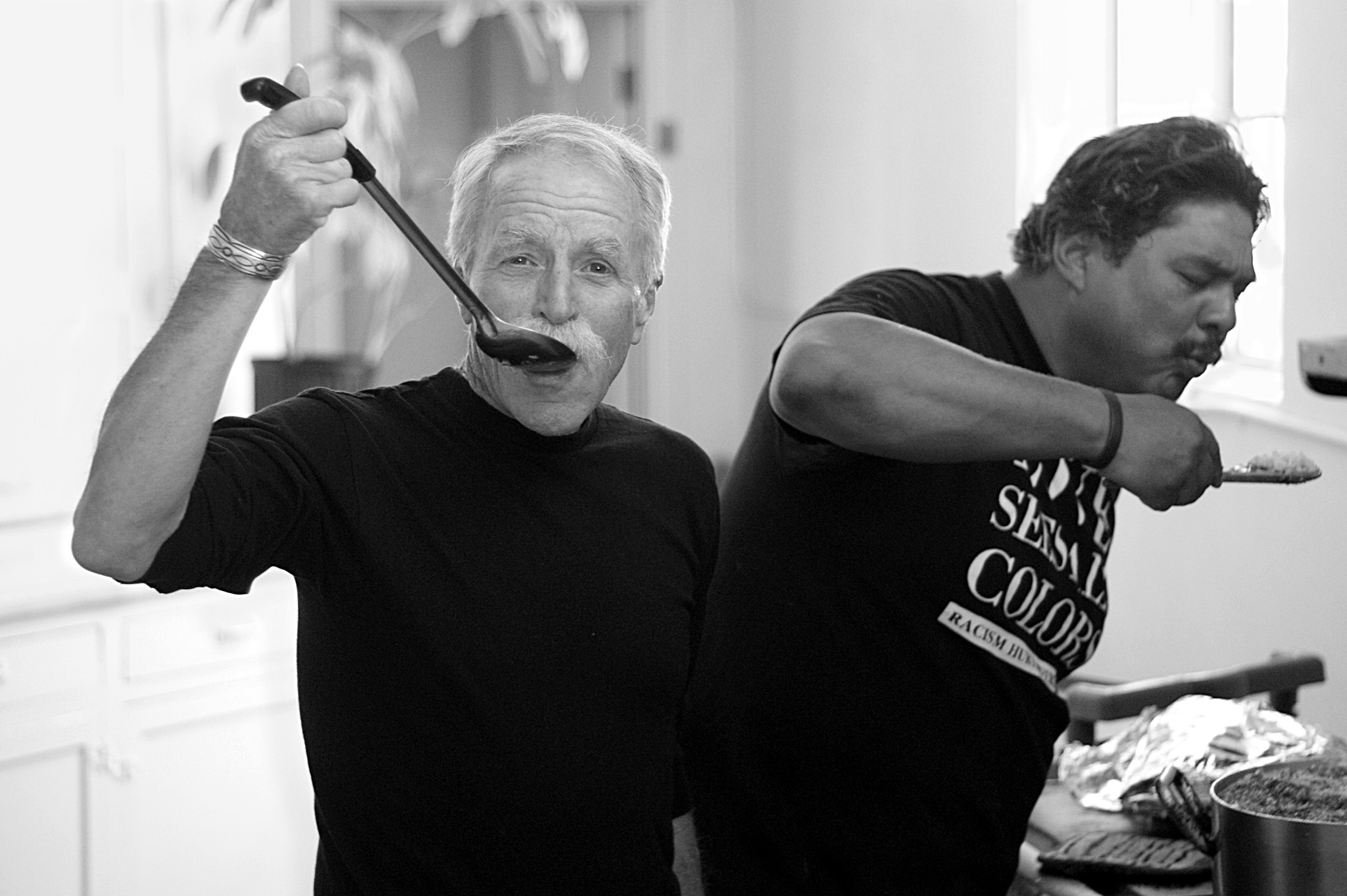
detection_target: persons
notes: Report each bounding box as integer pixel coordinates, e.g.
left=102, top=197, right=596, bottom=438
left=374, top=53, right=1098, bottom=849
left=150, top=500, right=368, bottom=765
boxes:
left=695, top=117, right=1269, bottom=896
left=71, top=64, right=723, bottom=894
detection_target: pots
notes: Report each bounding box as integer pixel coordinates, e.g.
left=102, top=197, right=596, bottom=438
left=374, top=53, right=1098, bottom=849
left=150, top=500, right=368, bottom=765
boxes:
left=1209, top=756, right=1347, bottom=896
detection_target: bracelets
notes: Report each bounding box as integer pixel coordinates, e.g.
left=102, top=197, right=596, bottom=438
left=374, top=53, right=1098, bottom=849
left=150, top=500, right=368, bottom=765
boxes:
left=205, top=219, right=288, bottom=281
left=1079, top=389, right=1123, bottom=468
